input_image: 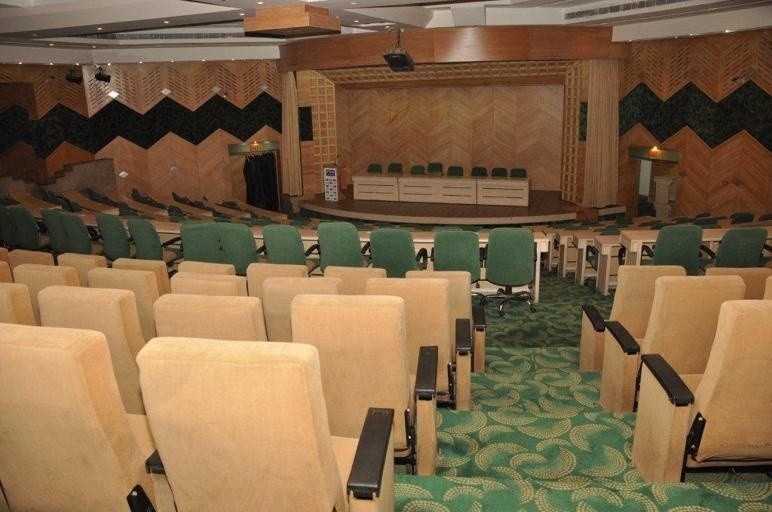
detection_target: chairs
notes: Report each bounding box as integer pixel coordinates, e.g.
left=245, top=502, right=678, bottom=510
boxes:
left=367, top=162, right=527, bottom=177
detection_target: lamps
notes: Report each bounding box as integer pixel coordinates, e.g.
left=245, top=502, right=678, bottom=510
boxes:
left=94, top=64, right=111, bottom=83
left=65, top=65, right=82, bottom=84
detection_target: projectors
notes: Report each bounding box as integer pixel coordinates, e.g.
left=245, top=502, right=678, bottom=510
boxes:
left=381, top=47, right=415, bottom=72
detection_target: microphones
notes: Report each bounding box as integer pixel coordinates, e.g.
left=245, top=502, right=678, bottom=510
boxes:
left=440, top=163, right=442, bottom=170
